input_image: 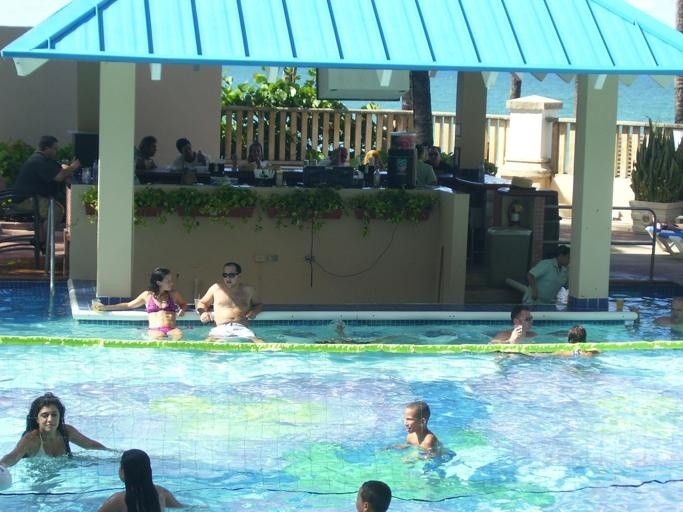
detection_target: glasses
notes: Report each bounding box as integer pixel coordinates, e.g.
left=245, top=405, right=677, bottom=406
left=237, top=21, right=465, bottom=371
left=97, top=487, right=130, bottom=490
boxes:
left=223, top=272, right=239, bottom=278
left=519, top=316, right=533, bottom=322
left=429, top=151, right=439, bottom=156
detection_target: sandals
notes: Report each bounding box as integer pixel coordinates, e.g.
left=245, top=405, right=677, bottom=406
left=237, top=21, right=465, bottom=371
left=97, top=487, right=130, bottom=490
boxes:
left=30, top=239, right=48, bottom=255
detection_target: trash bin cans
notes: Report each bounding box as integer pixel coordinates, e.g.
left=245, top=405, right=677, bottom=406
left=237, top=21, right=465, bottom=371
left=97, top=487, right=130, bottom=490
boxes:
left=486, top=225, right=532, bottom=279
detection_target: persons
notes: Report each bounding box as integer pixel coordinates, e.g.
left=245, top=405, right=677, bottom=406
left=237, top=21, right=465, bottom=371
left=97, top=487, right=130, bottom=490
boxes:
left=652, top=214, right=683, bottom=231
left=551, top=324, right=603, bottom=369
left=134, top=136, right=263, bottom=186
left=355, top=481, right=391, bottom=512
left=98, top=449, right=209, bottom=512
left=195, top=263, right=266, bottom=343
left=380, top=400, right=456, bottom=482
left=91, top=267, right=188, bottom=341
left=9, top=133, right=80, bottom=256
left=628, top=294, right=683, bottom=335
left=521, top=245, right=570, bottom=305
left=0, top=391, right=119, bottom=474
left=486, top=305, right=549, bottom=364
left=319, top=134, right=452, bottom=186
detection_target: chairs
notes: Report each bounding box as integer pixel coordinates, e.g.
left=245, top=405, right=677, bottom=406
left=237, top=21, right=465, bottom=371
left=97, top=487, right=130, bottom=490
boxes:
left=135, top=162, right=454, bottom=190
left=0, top=185, right=44, bottom=271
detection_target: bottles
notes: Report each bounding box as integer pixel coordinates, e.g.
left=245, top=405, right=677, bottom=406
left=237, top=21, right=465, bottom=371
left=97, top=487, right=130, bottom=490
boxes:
left=92, top=159, right=97, bottom=184
left=80, top=168, right=91, bottom=184
left=233, top=159, right=239, bottom=178
left=478, top=161, right=484, bottom=183
left=352, top=168, right=380, bottom=187
left=223, top=166, right=232, bottom=174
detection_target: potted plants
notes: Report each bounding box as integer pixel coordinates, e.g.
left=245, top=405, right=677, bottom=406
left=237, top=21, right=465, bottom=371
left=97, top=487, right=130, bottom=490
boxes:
left=623, top=112, right=683, bottom=236
left=79, top=186, right=442, bottom=228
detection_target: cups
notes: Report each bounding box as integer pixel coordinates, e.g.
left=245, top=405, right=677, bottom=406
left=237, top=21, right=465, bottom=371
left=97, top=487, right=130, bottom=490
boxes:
left=216, top=160, right=224, bottom=177
left=512, top=324, right=522, bottom=336
left=91, top=298, right=100, bottom=313
left=252, top=168, right=274, bottom=179
left=193, top=298, right=200, bottom=312
left=208, top=160, right=214, bottom=177
left=615, top=298, right=623, bottom=312
